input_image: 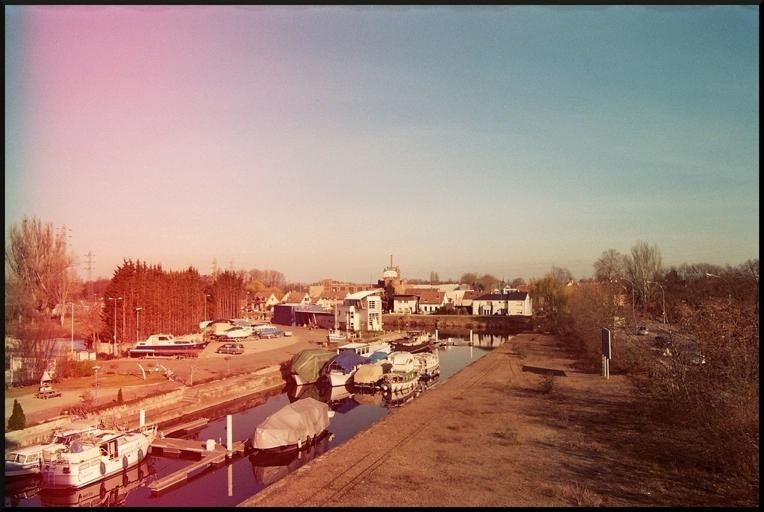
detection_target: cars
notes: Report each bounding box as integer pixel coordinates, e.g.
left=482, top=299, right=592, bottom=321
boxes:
left=611, top=316, right=626, bottom=327
left=207, top=318, right=283, bottom=339
left=34, top=389, right=61, bottom=400
left=633, top=325, right=706, bottom=366
left=217, top=342, right=244, bottom=355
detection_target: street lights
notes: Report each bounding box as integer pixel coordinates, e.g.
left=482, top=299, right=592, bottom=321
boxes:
left=135, top=307, right=144, bottom=342
left=619, top=277, right=635, bottom=323
left=108, top=297, right=122, bottom=357
left=704, top=273, right=732, bottom=354
left=91, top=364, right=99, bottom=406
left=646, top=279, right=665, bottom=325
left=203, top=293, right=210, bottom=337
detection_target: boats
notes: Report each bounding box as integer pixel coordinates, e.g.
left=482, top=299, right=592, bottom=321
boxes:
left=40, top=423, right=158, bottom=490
left=253, top=397, right=330, bottom=452
left=289, top=329, right=454, bottom=392
left=289, top=363, right=440, bottom=408
left=131, top=333, right=210, bottom=357
left=251, top=430, right=330, bottom=489
left=4, top=459, right=153, bottom=506
left=3, top=425, right=105, bottom=481
